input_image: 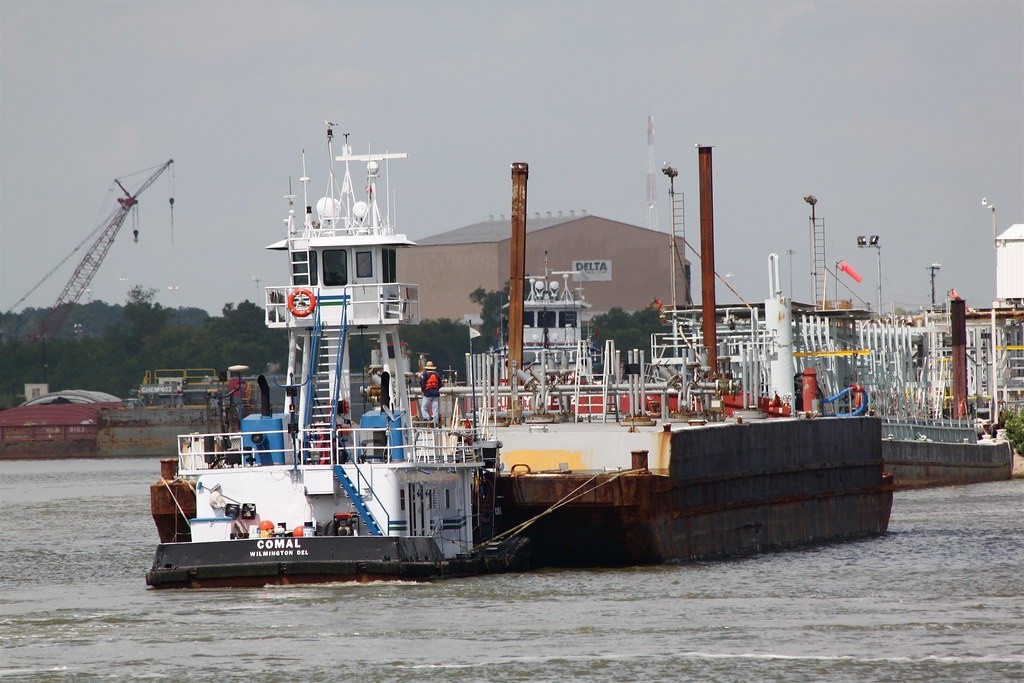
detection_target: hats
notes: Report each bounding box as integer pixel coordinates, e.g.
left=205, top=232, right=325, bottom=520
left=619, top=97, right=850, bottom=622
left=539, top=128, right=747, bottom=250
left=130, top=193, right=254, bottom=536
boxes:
left=422, top=361, right=436, bottom=369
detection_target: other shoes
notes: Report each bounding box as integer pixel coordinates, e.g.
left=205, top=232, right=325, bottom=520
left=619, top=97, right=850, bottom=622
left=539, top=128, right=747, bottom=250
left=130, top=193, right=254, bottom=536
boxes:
left=423, top=419, right=428, bottom=421
left=431, top=423, right=437, bottom=428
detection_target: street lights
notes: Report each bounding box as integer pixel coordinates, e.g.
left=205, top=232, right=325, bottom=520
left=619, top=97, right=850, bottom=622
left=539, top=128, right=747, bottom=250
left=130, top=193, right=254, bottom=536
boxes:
left=803, top=193, right=821, bottom=306
left=854, top=233, right=884, bottom=319
left=659, top=163, right=682, bottom=357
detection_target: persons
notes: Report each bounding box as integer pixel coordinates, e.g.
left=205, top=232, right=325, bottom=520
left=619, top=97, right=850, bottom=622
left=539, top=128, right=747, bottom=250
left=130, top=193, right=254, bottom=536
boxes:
left=420, top=361, right=443, bottom=426
left=177, top=382, right=186, bottom=405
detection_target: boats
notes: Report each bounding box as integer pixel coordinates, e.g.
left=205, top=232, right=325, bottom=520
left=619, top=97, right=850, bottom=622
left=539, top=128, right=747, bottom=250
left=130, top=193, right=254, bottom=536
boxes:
left=143, top=125, right=537, bottom=597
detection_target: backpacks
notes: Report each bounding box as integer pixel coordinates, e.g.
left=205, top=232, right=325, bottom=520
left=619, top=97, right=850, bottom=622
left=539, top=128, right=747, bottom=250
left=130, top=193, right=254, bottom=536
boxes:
left=425, top=374, right=439, bottom=390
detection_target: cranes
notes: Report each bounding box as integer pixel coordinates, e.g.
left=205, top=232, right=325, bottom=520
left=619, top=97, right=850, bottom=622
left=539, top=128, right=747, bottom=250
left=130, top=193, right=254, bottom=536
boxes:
left=4, top=154, right=178, bottom=342
